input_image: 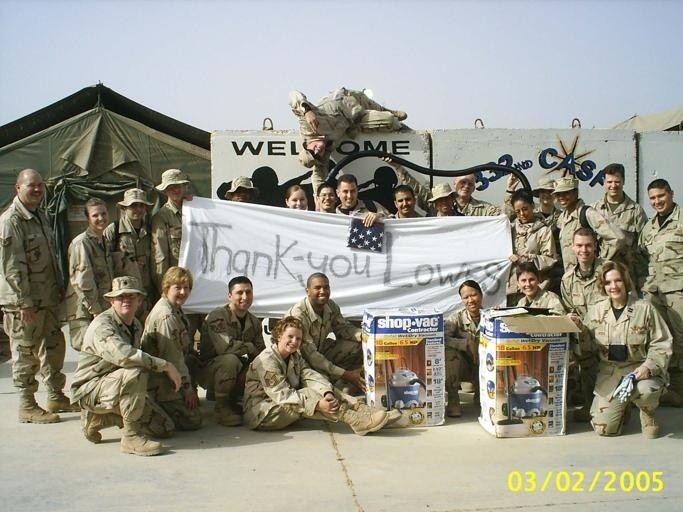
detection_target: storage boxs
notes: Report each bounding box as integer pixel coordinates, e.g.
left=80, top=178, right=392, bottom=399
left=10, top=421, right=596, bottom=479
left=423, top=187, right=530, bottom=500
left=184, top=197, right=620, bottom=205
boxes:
left=475, top=305, right=581, bottom=438
left=363, top=303, right=446, bottom=429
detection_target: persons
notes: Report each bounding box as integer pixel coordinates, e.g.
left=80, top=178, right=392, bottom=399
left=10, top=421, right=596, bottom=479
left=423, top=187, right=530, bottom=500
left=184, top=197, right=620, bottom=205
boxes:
left=69, top=277, right=182, bottom=456
left=104, top=187, right=154, bottom=316
left=289, top=85, right=412, bottom=212
left=140, top=266, right=208, bottom=430
left=66, top=196, right=113, bottom=352
left=0, top=169, right=83, bottom=424
left=148, top=168, right=193, bottom=298
left=191, top=157, right=683, bottom=439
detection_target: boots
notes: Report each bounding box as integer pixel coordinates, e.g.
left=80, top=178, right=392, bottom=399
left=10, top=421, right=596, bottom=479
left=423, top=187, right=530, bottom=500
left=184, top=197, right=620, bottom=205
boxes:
left=204, top=402, right=244, bottom=430
left=639, top=406, right=664, bottom=442
left=378, top=104, right=409, bottom=122
left=346, top=402, right=403, bottom=435
left=16, top=395, right=163, bottom=456
left=446, top=386, right=465, bottom=422
left=658, top=368, right=683, bottom=408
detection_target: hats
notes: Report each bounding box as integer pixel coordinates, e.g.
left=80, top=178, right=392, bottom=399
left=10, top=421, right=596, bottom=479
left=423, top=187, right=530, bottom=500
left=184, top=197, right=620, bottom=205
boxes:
left=296, top=141, right=326, bottom=170
left=116, top=188, right=154, bottom=209
left=102, top=277, right=148, bottom=302
left=531, top=176, right=582, bottom=198
left=155, top=168, right=190, bottom=191
left=222, top=175, right=265, bottom=200
left=427, top=181, right=460, bottom=207
